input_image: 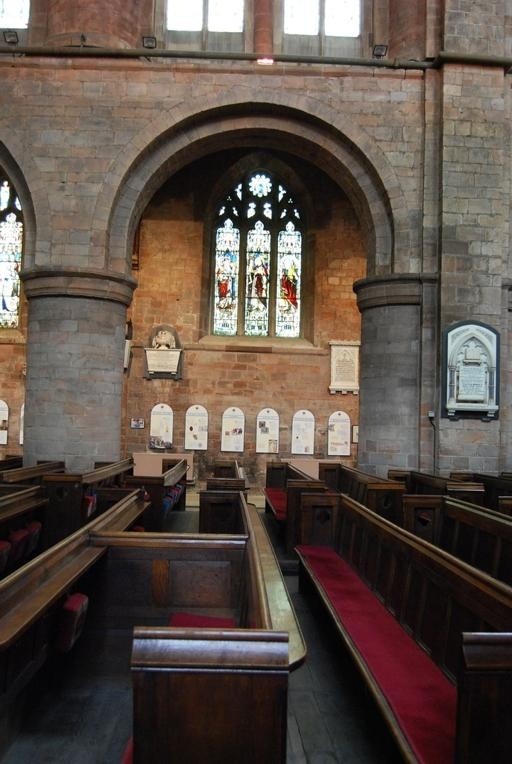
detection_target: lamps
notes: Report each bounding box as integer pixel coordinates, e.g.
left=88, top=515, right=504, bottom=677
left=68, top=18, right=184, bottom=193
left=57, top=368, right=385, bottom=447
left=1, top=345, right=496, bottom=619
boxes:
left=143, top=35, right=156, bottom=50
left=3, top=28, right=19, bottom=45
left=372, top=44, right=388, bottom=59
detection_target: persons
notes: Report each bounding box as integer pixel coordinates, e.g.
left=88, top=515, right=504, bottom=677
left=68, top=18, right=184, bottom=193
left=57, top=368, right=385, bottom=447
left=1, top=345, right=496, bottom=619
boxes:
left=246, top=256, right=269, bottom=312
left=279, top=257, right=299, bottom=311
left=217, top=256, right=238, bottom=310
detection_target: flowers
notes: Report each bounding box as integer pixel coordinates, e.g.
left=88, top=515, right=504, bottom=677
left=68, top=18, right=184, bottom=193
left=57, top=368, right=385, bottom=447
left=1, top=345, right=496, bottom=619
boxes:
left=147, top=435, right=172, bottom=448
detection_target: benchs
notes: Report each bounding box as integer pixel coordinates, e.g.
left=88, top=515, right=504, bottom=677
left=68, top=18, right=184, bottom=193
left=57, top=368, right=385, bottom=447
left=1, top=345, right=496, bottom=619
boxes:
left=0, top=457, right=512, bottom=764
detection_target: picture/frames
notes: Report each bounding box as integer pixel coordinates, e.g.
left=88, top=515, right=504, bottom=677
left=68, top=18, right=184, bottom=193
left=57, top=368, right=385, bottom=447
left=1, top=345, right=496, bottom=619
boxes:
left=439, top=318, right=501, bottom=423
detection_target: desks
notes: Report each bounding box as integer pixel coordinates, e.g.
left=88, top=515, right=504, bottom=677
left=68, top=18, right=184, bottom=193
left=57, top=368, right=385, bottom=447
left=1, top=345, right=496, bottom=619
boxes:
left=132, top=449, right=196, bottom=485
